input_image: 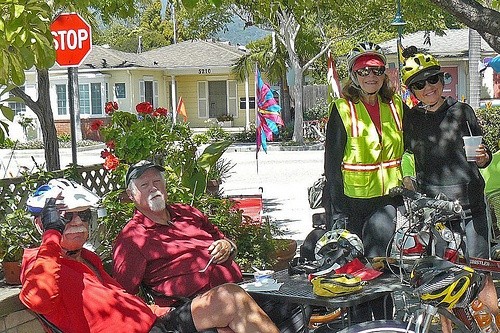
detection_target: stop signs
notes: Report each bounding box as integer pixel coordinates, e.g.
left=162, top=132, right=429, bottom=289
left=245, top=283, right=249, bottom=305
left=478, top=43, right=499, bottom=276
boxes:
left=49, top=13, right=92, bottom=69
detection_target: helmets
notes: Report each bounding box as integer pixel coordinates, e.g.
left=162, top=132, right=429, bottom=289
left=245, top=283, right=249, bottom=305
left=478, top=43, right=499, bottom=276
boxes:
left=314, top=228, right=365, bottom=267
left=26, top=178, right=101, bottom=215
left=346, top=42, right=386, bottom=70
left=401, top=53, right=440, bottom=84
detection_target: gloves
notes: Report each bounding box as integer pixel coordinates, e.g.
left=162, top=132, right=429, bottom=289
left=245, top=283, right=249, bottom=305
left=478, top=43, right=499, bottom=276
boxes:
left=41, top=198, right=69, bottom=235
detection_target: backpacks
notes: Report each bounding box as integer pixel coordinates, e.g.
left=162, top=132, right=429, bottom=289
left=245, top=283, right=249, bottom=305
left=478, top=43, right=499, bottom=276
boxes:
left=310, top=273, right=365, bottom=297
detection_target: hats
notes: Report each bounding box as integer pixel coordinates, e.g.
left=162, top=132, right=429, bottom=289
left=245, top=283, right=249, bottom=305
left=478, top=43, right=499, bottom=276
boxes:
left=352, top=55, right=384, bottom=72
left=408, top=70, right=444, bottom=84
left=126, top=160, right=166, bottom=186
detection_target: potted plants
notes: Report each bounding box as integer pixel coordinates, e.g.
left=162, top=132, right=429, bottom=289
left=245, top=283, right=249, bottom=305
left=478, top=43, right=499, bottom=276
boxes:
left=0, top=209, right=42, bottom=285
left=218, top=114, right=234, bottom=128
left=236, top=216, right=297, bottom=267
left=207, top=159, right=237, bottom=194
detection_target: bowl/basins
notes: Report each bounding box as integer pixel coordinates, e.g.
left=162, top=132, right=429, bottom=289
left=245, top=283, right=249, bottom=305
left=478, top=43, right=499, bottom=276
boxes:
left=254, top=269, right=276, bottom=286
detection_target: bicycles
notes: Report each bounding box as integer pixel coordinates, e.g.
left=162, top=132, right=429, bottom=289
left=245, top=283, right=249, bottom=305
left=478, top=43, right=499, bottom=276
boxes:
left=337, top=187, right=500, bottom=333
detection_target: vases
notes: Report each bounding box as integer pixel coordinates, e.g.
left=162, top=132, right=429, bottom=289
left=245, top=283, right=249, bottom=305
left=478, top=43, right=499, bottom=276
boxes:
left=120, top=191, right=133, bottom=203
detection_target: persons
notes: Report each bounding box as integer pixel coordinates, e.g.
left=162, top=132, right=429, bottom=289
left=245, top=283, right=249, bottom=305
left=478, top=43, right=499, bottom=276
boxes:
left=400, top=53, right=500, bottom=333
left=321, top=42, right=411, bottom=322
left=111, top=160, right=244, bottom=306
left=19, top=178, right=281, bottom=333
left=480, top=150, right=500, bottom=231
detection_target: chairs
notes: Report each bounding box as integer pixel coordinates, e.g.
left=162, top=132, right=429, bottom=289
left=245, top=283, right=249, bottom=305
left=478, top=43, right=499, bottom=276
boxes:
left=486, top=190, right=500, bottom=284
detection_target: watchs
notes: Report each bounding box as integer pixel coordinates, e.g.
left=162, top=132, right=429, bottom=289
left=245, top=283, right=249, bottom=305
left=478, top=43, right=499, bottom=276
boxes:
left=228, top=241, right=236, bottom=256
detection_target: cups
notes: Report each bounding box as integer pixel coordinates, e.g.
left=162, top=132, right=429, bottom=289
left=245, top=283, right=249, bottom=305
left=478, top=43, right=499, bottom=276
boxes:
left=462, top=136, right=482, bottom=161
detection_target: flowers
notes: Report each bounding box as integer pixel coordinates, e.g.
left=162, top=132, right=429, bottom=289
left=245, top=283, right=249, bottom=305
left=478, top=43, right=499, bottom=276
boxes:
left=90, top=102, right=193, bottom=188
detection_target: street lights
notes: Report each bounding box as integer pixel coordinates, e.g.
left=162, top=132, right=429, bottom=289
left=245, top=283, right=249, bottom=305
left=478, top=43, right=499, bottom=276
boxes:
left=391, top=0, right=407, bottom=95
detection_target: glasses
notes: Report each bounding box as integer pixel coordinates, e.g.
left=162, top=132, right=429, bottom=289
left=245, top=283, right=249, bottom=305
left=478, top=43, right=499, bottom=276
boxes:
left=412, top=73, right=441, bottom=89
left=337, top=237, right=362, bottom=258
left=61, top=208, right=92, bottom=223
left=356, top=67, right=384, bottom=77
left=288, top=257, right=307, bottom=276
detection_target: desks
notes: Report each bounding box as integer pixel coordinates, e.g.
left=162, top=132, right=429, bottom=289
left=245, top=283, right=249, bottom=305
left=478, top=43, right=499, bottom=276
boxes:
left=236, top=269, right=410, bottom=333
